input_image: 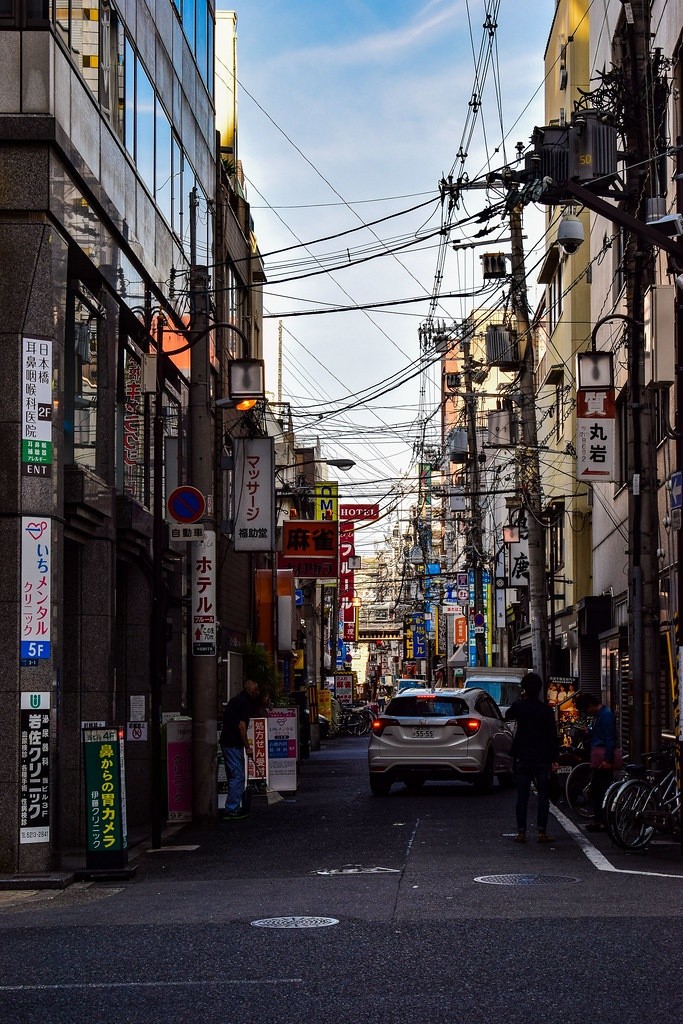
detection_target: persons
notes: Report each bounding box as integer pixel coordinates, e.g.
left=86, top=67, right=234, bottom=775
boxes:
left=547, top=683, right=575, bottom=707
left=574, top=693, right=621, bottom=833
left=216, top=675, right=259, bottom=819
left=503, top=672, right=559, bottom=841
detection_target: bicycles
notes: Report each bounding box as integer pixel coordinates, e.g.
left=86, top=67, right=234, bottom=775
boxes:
left=326, top=701, right=380, bottom=737
left=565, top=731, right=683, bottom=853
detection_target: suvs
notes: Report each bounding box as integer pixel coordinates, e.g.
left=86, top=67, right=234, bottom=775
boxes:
left=365, top=686, right=516, bottom=789
left=463, top=674, right=522, bottom=732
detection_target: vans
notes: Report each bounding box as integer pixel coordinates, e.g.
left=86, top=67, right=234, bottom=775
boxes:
left=394, top=679, right=428, bottom=695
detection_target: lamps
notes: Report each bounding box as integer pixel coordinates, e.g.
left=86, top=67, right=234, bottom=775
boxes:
left=157, top=313, right=266, bottom=400
left=211, top=396, right=257, bottom=410
left=275, top=460, right=356, bottom=473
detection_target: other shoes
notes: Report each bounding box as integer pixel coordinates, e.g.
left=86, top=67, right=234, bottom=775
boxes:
left=536, top=835, right=557, bottom=843
left=223, top=809, right=249, bottom=821
left=513, top=836, right=526, bottom=843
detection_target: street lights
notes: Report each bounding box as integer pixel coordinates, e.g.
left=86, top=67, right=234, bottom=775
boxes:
left=147, top=317, right=263, bottom=847
left=273, top=457, right=356, bottom=689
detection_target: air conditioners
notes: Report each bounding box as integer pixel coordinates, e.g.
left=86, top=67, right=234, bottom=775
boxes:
left=448, top=487, right=465, bottom=511
left=449, top=428, right=469, bottom=453
left=488, top=411, right=510, bottom=445
